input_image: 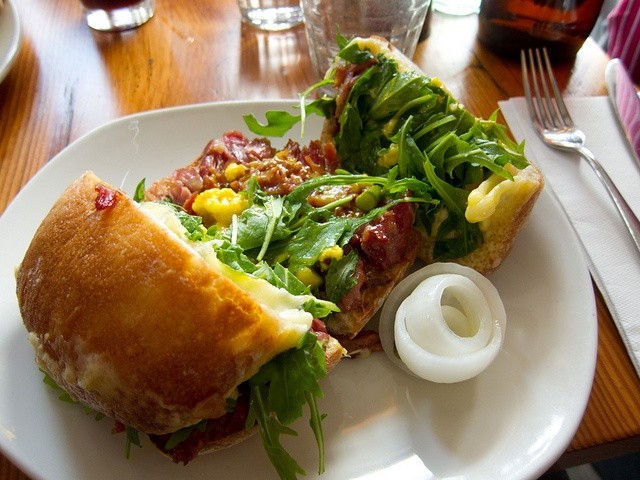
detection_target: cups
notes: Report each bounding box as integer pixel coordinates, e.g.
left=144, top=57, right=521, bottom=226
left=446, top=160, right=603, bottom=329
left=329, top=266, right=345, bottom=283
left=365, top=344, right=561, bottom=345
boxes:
left=77, top=1, right=156, bottom=30
left=298, top=1, right=432, bottom=96
left=239, top=1, right=302, bottom=31
left=478, top=1, right=604, bottom=67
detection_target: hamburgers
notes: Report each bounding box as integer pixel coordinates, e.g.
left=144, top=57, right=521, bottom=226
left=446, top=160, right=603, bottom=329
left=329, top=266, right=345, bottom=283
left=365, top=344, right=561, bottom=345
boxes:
left=15, top=32, right=545, bottom=480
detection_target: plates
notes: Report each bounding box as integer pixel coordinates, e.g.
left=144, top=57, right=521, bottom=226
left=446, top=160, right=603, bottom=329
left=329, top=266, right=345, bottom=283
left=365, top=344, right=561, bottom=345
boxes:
left=0, top=0, right=21, bottom=83
left=1, top=100, right=598, bottom=480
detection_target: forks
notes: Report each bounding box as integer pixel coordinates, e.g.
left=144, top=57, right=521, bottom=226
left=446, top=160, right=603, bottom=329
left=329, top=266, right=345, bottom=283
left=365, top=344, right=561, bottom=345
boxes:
left=521, top=47, right=640, bottom=251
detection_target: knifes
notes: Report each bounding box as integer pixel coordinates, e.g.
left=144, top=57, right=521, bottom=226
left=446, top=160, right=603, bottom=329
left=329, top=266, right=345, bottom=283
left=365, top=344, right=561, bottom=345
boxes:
left=604, top=58, right=639, bottom=165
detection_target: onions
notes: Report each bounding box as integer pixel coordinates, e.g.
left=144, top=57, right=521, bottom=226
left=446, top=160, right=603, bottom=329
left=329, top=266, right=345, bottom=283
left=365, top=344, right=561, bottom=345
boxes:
left=405, top=274, right=492, bottom=355
left=377, top=262, right=506, bottom=380
left=393, top=291, right=501, bottom=382
